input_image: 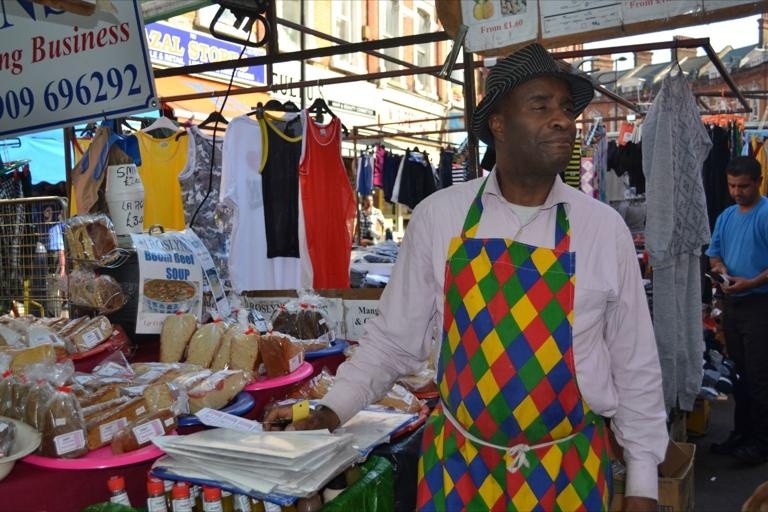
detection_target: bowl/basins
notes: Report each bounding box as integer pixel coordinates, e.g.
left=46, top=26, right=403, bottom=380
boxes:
left=143, top=278, right=198, bottom=313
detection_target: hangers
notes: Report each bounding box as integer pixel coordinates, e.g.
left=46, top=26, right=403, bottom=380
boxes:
left=81, top=79, right=349, bottom=141
left=587, top=62, right=695, bottom=147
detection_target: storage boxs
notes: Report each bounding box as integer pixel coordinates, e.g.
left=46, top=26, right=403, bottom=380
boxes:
left=81, top=455, right=395, bottom=512
left=611, top=441, right=696, bottom=512
left=687, top=399, right=712, bottom=435
left=369, top=423, right=426, bottom=512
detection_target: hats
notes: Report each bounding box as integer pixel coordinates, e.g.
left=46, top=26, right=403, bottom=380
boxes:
left=470, top=42, right=594, bottom=144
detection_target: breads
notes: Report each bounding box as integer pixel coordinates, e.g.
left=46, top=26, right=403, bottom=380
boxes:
left=0, top=215, right=436, bottom=460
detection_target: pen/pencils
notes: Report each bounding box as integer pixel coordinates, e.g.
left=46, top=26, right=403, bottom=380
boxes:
left=262, top=420, right=292, bottom=425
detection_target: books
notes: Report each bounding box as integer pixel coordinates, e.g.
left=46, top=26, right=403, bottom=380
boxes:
left=147, top=402, right=421, bottom=497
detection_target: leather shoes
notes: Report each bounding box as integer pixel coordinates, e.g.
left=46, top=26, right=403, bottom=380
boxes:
left=725, top=431, right=768, bottom=464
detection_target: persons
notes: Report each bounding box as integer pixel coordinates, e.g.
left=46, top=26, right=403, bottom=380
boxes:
left=352, top=195, right=387, bottom=246
left=702, top=154, right=768, bottom=468
left=258, top=42, right=672, bottom=511
left=47, top=220, right=69, bottom=280
